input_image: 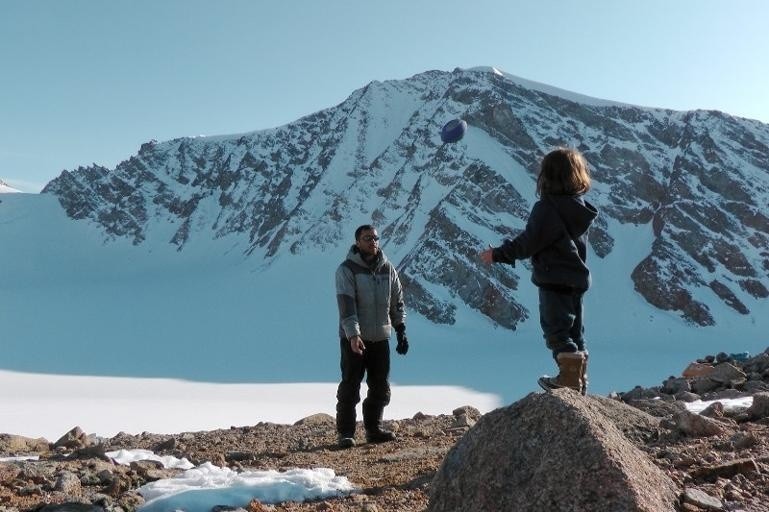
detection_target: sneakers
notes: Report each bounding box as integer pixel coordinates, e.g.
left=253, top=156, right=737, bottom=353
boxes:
left=338, top=433, right=355, bottom=449
left=366, top=426, right=396, bottom=443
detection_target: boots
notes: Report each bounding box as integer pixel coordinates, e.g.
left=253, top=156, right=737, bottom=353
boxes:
left=538, top=351, right=589, bottom=397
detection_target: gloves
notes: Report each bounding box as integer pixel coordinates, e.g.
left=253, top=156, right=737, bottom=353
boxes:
left=395, top=322, right=408, bottom=355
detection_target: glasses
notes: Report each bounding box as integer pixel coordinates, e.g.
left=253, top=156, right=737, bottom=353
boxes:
left=357, top=234, right=380, bottom=242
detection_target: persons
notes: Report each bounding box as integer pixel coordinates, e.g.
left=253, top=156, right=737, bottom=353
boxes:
left=335, top=225, right=409, bottom=448
left=479, top=149, right=599, bottom=396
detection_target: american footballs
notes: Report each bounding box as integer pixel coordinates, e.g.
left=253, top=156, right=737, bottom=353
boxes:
left=442, top=119, right=467, bottom=143
